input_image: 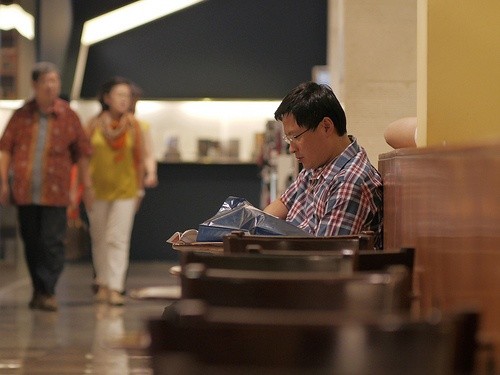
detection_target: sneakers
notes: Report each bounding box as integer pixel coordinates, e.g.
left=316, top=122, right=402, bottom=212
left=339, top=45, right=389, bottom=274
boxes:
left=28, top=296, right=41, bottom=310
left=40, top=295, right=58, bottom=310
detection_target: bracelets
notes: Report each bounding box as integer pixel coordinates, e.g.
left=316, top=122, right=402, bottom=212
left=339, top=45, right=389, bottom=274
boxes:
left=82, top=185, right=92, bottom=189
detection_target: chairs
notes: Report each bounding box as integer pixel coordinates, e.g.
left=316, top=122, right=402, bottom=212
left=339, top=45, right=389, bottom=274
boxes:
left=148, top=231, right=480, bottom=374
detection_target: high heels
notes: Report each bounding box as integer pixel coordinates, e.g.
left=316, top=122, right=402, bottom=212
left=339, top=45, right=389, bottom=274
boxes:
left=108, top=289, right=124, bottom=305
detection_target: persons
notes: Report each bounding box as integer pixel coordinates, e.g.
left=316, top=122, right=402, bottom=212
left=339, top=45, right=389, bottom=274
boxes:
left=77, top=78, right=158, bottom=305
left=0, top=61, right=95, bottom=311
left=264, top=82, right=383, bottom=251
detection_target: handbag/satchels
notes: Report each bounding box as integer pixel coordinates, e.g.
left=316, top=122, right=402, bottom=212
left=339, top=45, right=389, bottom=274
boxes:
left=196, top=195, right=314, bottom=242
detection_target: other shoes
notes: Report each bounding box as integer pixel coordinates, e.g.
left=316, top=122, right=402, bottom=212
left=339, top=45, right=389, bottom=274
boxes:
left=93, top=295, right=109, bottom=304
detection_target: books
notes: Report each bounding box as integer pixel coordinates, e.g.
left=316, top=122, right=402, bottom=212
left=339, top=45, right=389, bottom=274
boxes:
left=166, top=229, right=198, bottom=245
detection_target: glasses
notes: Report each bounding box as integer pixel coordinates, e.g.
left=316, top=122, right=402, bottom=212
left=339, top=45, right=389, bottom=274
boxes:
left=282, top=115, right=329, bottom=144
